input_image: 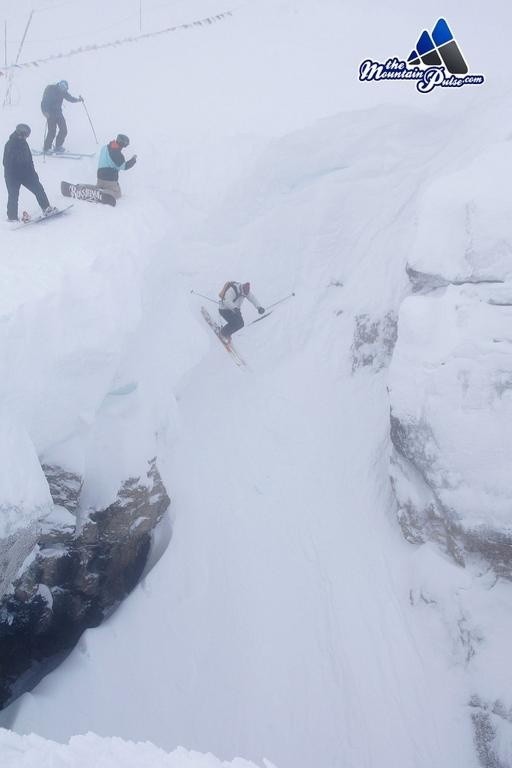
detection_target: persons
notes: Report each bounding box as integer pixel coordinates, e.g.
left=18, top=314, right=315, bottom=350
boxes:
left=217, top=280, right=268, bottom=346
left=3, top=123, right=59, bottom=224
left=75, top=133, right=139, bottom=199
left=41, top=78, right=85, bottom=156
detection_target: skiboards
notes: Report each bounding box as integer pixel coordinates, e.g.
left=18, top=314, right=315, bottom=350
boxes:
left=30, top=149, right=96, bottom=160
left=200, top=305, right=250, bottom=374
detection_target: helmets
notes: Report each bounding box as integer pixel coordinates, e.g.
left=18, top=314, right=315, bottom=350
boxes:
left=116, top=134, right=129, bottom=145
left=17, top=124, right=31, bottom=136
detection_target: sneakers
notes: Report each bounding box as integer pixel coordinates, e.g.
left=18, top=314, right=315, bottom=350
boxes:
left=216, top=326, right=232, bottom=344
left=42, top=207, right=59, bottom=216
left=7, top=217, right=19, bottom=222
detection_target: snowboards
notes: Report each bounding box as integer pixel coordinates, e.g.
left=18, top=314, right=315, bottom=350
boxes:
left=10, top=204, right=74, bottom=231
left=61, top=181, right=116, bottom=208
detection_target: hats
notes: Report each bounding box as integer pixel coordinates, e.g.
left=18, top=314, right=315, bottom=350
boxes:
left=242, top=282, right=250, bottom=296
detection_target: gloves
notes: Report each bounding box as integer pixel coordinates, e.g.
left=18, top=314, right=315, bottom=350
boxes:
left=233, top=308, right=240, bottom=313
left=258, top=307, right=265, bottom=314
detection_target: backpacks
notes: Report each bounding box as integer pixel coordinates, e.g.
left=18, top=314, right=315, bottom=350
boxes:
left=219, top=280, right=237, bottom=299
